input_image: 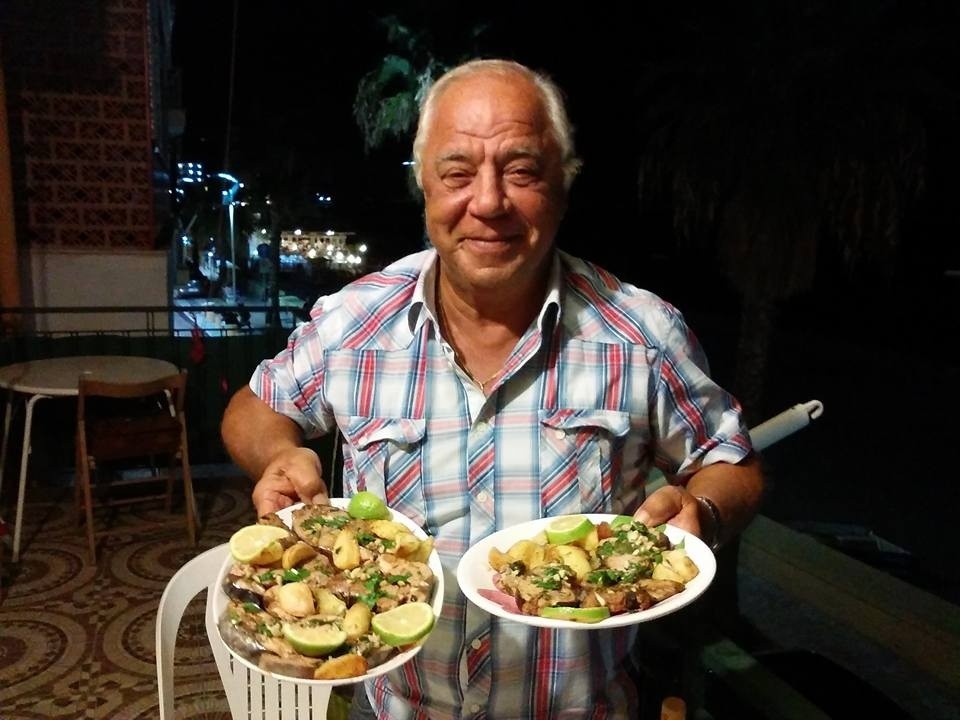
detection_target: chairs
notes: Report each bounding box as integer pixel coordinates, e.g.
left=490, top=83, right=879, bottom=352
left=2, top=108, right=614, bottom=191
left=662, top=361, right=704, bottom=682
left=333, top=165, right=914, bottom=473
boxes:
left=76, top=369, right=202, bottom=563
left=154, top=543, right=333, bottom=720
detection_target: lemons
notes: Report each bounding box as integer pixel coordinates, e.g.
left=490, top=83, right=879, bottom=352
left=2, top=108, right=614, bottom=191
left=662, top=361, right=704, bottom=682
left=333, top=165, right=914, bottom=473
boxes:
left=347, top=491, right=390, bottom=520
left=372, top=601, right=436, bottom=647
left=543, top=515, right=594, bottom=544
left=541, top=606, right=611, bottom=621
left=281, top=622, right=347, bottom=656
left=229, top=524, right=290, bottom=562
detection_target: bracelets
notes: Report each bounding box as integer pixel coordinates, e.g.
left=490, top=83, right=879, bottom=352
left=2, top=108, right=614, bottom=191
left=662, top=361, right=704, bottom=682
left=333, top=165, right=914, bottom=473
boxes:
left=695, top=497, right=721, bottom=552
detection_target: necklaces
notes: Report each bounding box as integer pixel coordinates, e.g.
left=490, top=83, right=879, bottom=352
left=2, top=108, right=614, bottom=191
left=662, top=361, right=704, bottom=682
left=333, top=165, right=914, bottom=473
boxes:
left=437, top=292, right=502, bottom=390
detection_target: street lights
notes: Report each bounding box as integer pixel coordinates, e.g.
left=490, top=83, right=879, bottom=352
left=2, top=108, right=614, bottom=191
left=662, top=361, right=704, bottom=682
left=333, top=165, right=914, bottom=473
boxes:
left=217, top=173, right=240, bottom=301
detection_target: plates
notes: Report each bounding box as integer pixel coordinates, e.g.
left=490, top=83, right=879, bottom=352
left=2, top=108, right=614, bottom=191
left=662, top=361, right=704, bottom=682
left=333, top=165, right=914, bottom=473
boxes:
left=211, top=498, right=444, bottom=684
left=455, top=513, right=718, bottom=630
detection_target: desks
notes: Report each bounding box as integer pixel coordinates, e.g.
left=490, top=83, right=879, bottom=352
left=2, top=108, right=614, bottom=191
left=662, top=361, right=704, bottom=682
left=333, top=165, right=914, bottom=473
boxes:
left=0, top=355, right=179, bottom=563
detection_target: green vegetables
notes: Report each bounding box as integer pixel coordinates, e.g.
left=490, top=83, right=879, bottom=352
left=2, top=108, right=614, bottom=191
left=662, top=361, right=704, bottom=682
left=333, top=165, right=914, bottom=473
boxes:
left=224, top=514, right=412, bottom=637
left=529, top=516, right=670, bottom=591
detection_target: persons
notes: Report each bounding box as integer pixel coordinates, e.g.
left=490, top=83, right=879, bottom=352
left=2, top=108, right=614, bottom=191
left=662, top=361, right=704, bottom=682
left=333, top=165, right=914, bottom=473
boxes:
left=221, top=59, right=767, bottom=720
